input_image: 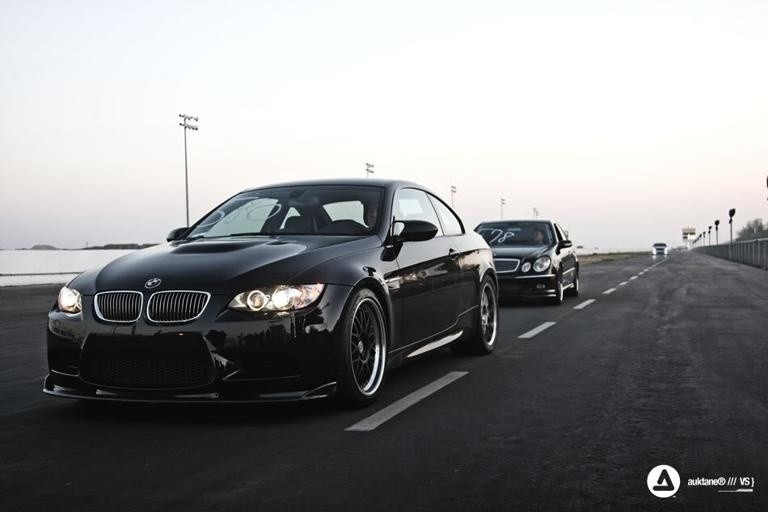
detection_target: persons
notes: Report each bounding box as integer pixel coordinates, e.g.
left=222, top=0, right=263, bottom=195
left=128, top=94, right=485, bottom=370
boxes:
left=366, top=199, right=380, bottom=227
left=531, top=229, right=545, bottom=243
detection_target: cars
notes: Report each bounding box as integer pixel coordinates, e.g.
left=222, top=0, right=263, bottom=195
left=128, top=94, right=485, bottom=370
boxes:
left=41, top=180, right=500, bottom=406
left=473, top=220, right=580, bottom=305
left=651, top=241, right=669, bottom=256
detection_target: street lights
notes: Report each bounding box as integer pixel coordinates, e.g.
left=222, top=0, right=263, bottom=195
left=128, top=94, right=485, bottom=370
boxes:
left=364, top=160, right=376, bottom=179
left=450, top=184, right=457, bottom=208
left=177, top=112, right=199, bottom=229
left=690, top=208, right=736, bottom=259
left=500, top=198, right=506, bottom=221
